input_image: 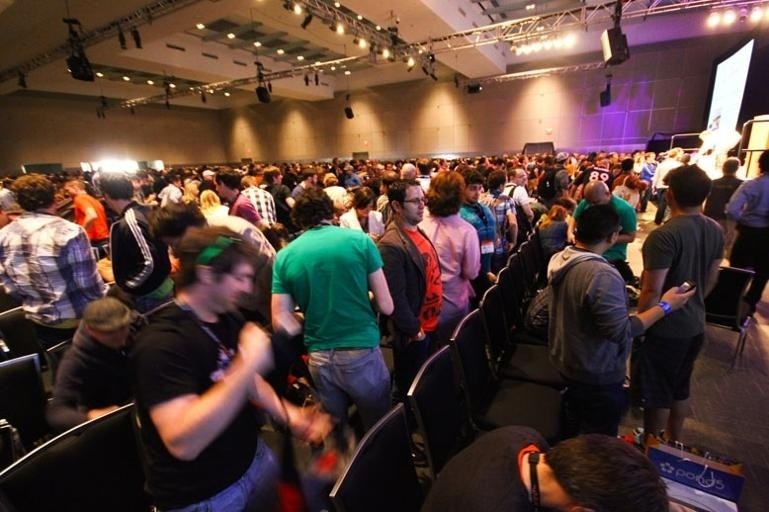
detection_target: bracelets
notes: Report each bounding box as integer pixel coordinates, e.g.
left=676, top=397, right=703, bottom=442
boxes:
left=656, top=297, right=675, bottom=318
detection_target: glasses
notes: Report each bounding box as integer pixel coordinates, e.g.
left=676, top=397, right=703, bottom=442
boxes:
left=402, top=196, right=427, bottom=206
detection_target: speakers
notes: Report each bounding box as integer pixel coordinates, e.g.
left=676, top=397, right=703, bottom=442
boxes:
left=601, top=28, right=628, bottom=66
left=67, top=56, right=95, bottom=81
left=256, top=87, right=271, bottom=103
left=344, top=107, right=354, bottom=119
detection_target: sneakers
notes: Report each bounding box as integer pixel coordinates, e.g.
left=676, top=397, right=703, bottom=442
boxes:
left=631, top=426, right=667, bottom=449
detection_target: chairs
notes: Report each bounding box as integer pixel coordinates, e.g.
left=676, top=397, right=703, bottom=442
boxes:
left=702, top=263, right=755, bottom=371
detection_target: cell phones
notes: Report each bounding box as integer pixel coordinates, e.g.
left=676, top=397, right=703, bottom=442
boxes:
left=680, top=278, right=695, bottom=292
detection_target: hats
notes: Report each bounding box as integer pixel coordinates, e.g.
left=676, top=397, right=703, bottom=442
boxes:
left=556, top=151, right=570, bottom=163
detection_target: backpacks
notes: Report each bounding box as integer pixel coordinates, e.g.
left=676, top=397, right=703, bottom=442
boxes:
left=544, top=168, right=566, bottom=199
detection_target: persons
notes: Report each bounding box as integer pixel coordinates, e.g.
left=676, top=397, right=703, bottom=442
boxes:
left=0, top=145, right=769, bottom=339
left=638, top=166, right=725, bottom=449
left=267, top=189, right=397, bottom=431
left=47, top=297, right=149, bottom=431
left=131, top=227, right=336, bottom=512
left=376, top=177, right=443, bottom=395
left=415, top=426, right=668, bottom=510
left=548, top=206, right=700, bottom=447
